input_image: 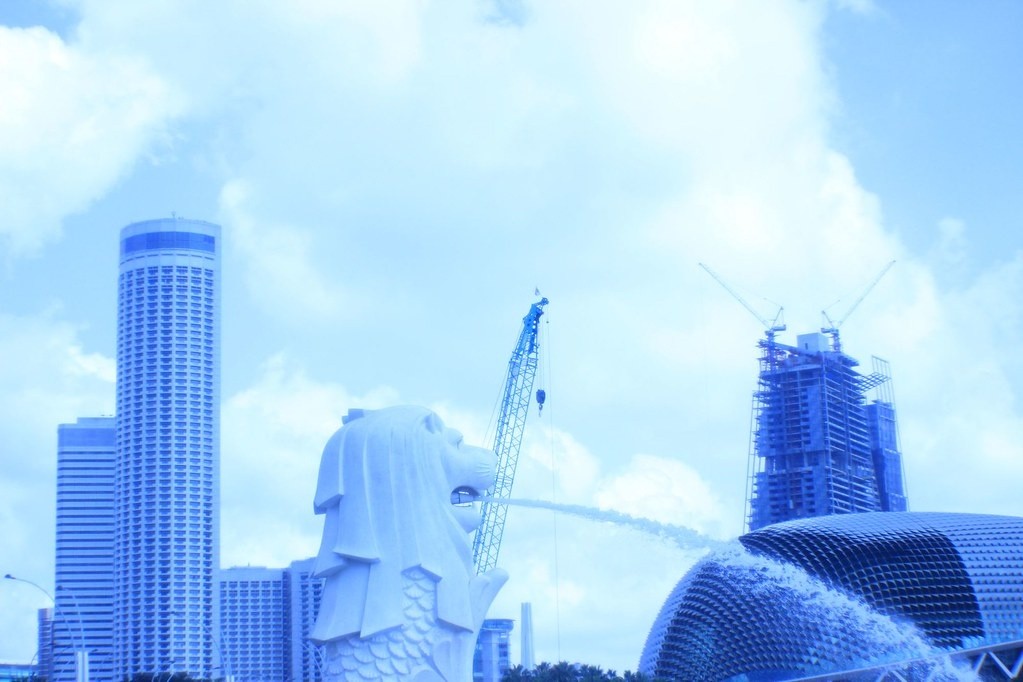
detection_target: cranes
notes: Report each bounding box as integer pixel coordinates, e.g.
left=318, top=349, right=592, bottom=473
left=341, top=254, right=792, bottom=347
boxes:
left=699, top=262, right=789, bottom=366
left=471, top=298, right=552, bottom=578
left=821, top=259, right=897, bottom=351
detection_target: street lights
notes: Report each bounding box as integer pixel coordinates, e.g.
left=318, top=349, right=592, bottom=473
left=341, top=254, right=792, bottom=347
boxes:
left=3, top=575, right=88, bottom=682
